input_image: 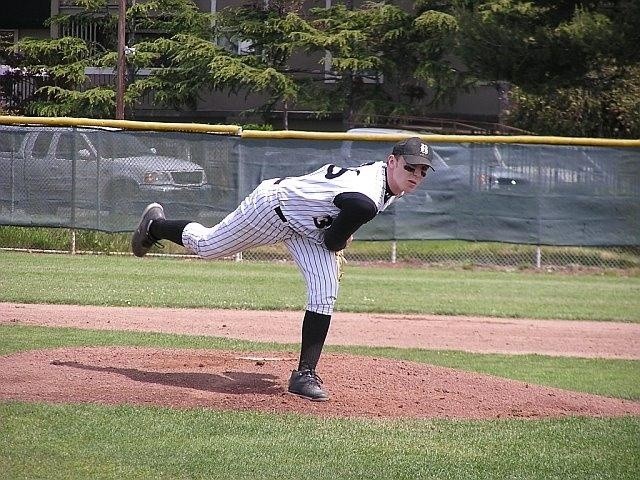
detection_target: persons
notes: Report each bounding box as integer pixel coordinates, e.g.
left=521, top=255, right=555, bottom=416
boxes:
left=130, top=136, right=436, bottom=403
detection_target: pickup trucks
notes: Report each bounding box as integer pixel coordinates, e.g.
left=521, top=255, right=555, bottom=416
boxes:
left=0, top=124, right=207, bottom=213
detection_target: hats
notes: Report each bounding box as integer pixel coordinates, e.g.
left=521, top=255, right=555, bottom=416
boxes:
left=391, top=136, right=437, bottom=172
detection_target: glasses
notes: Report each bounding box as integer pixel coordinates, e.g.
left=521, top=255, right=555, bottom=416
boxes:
left=399, top=156, right=427, bottom=178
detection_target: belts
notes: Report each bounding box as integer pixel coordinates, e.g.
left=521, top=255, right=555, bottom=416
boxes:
left=271, top=175, right=288, bottom=227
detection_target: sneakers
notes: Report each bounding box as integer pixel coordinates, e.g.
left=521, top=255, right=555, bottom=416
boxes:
left=130, top=201, right=167, bottom=259
left=287, top=365, right=331, bottom=402
